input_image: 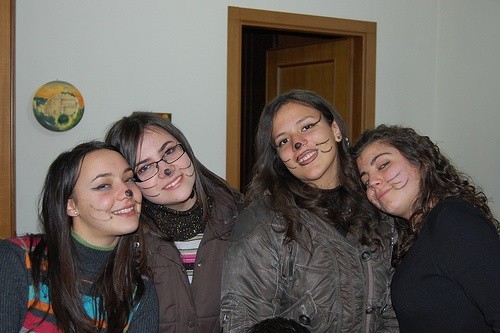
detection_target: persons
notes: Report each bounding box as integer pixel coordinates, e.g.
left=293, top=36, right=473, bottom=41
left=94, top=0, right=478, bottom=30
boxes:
left=351, top=124, right=500, bottom=333
left=0, top=141, right=159, bottom=333
left=105, top=112, right=246, bottom=333
left=219, top=89, right=400, bottom=333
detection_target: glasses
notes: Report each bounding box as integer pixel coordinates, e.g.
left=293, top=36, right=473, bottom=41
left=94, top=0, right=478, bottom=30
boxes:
left=133, top=143, right=186, bottom=182
